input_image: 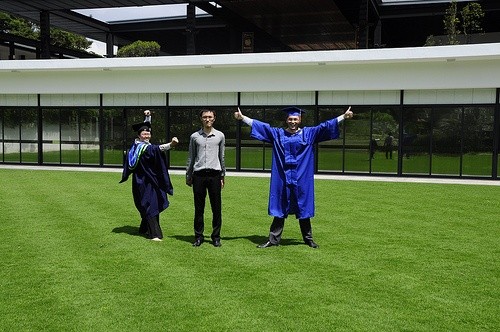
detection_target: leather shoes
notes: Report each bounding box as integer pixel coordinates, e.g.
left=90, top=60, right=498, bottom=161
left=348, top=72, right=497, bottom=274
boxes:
left=213, top=240, right=221, bottom=247
left=193, top=238, right=204, bottom=246
left=305, top=240, right=319, bottom=248
left=257, top=239, right=275, bottom=248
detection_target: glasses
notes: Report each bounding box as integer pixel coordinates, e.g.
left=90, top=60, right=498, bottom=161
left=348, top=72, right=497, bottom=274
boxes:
left=287, top=118, right=300, bottom=122
left=201, top=116, right=215, bottom=122
left=141, top=133, right=151, bottom=137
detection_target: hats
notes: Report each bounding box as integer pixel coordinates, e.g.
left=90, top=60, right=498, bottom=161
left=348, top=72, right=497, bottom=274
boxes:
left=132, top=121, right=152, bottom=133
left=281, top=107, right=306, bottom=123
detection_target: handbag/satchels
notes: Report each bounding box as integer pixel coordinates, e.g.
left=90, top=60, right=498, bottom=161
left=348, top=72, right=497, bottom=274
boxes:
left=391, top=138, right=399, bottom=147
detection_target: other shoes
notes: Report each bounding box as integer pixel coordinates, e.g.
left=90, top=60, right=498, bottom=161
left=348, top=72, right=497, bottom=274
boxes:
left=151, top=237, right=163, bottom=242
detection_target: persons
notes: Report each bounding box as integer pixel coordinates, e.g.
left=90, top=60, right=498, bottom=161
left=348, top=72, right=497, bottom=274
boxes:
left=234, top=106, right=353, bottom=248
left=119, top=110, right=179, bottom=241
left=186, top=107, right=226, bottom=247
left=384, top=131, right=394, bottom=159
left=369, top=135, right=378, bottom=159
